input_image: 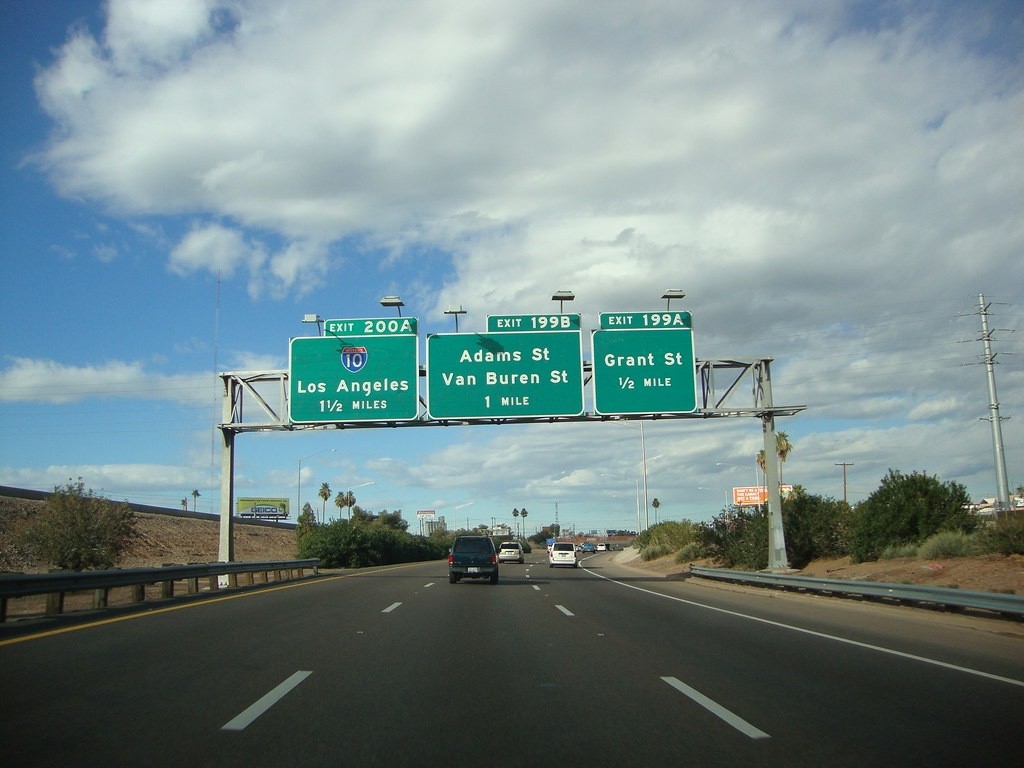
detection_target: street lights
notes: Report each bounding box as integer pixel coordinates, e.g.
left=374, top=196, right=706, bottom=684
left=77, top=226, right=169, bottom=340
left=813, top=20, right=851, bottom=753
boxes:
left=297, top=449, right=337, bottom=523
left=716, top=461, right=760, bottom=512
left=346, top=481, right=376, bottom=524
left=601, top=420, right=663, bottom=538
left=454, top=502, right=475, bottom=532
left=699, top=489, right=728, bottom=520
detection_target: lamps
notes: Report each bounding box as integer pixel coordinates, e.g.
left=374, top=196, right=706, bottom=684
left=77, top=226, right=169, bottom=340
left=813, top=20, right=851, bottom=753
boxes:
left=444, top=305, right=468, bottom=332
left=380, top=296, right=404, bottom=317
left=552, top=291, right=575, bottom=314
left=661, top=289, right=685, bottom=311
left=302, top=314, right=324, bottom=336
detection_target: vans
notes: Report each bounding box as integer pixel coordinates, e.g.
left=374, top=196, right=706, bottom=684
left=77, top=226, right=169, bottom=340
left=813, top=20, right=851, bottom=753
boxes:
left=549, top=542, right=578, bottom=568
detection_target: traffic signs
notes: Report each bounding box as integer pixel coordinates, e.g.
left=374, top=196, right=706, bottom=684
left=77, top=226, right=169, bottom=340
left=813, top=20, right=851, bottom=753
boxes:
left=425, top=313, right=586, bottom=419
left=288, top=315, right=419, bottom=422
left=588, top=313, right=698, bottom=419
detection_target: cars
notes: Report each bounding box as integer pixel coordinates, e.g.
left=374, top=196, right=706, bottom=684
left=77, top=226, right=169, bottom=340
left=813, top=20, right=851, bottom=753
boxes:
left=576, top=542, right=606, bottom=554
left=548, top=545, right=552, bottom=552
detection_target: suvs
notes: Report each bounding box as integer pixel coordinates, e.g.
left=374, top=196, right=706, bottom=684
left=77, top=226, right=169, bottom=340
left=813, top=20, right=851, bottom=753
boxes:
left=448, top=535, right=501, bottom=584
left=499, top=542, right=525, bottom=563
